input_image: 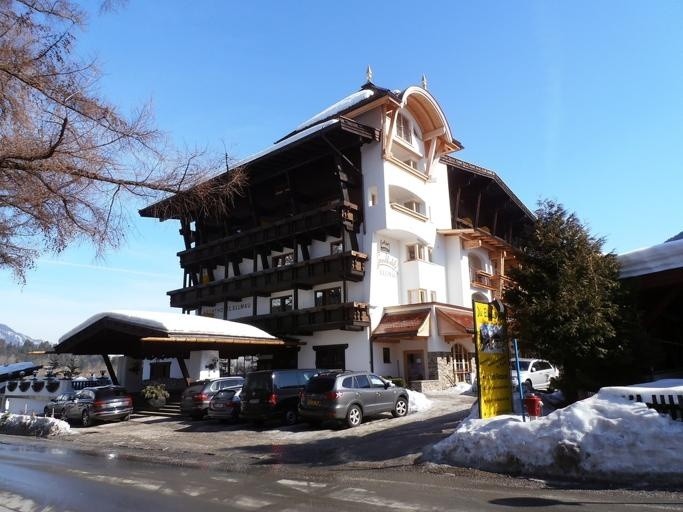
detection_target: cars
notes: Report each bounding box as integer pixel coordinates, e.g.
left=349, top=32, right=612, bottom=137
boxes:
left=180, top=369, right=409, bottom=428
left=44, top=385, right=133, bottom=427
left=510, top=358, right=559, bottom=394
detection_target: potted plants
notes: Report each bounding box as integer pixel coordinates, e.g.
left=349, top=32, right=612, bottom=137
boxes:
left=141, top=383, right=173, bottom=408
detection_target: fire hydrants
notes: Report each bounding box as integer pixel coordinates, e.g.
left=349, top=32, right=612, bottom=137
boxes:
left=523, top=393, right=544, bottom=417
